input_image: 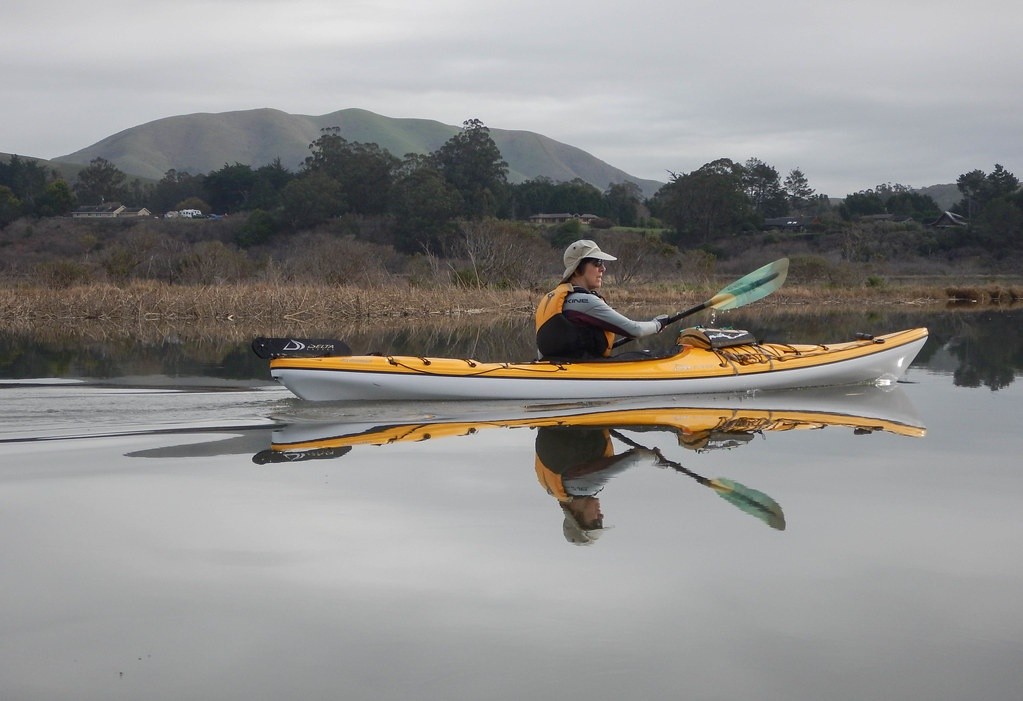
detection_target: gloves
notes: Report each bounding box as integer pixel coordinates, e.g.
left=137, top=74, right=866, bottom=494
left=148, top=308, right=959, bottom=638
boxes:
left=652, top=314, right=670, bottom=335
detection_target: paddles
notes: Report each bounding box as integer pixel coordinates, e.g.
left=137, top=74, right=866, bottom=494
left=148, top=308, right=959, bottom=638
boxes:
left=614, top=257, right=789, bottom=343
left=607, top=429, right=785, bottom=531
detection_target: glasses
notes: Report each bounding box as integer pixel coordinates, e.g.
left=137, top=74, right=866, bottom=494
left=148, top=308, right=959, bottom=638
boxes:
left=587, top=519, right=602, bottom=528
left=582, top=259, right=602, bottom=267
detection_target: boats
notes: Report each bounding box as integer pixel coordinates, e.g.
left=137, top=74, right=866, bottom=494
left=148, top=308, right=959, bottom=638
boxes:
left=268, top=328, right=929, bottom=401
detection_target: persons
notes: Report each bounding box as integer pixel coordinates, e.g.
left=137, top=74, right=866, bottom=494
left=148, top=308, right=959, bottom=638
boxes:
left=535, top=239, right=670, bottom=362
left=535, top=427, right=671, bottom=546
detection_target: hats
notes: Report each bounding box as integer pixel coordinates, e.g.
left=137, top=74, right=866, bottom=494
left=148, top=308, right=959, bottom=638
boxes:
left=558, top=240, right=617, bottom=284
left=559, top=502, right=615, bottom=547
